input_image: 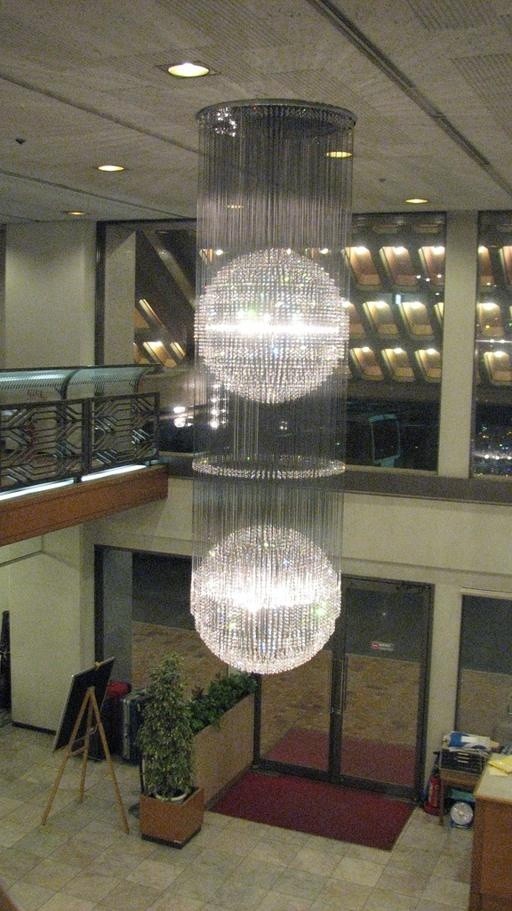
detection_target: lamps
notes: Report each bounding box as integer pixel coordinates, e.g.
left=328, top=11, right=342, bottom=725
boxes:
left=190, top=97, right=356, bottom=676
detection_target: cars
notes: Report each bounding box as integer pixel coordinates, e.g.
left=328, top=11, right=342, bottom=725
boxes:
left=346, top=408, right=512, bottom=476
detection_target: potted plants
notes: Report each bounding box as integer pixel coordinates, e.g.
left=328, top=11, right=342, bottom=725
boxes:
left=138, top=652, right=205, bottom=849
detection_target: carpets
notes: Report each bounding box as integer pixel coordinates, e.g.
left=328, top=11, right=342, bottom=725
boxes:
left=207, top=727, right=420, bottom=854
